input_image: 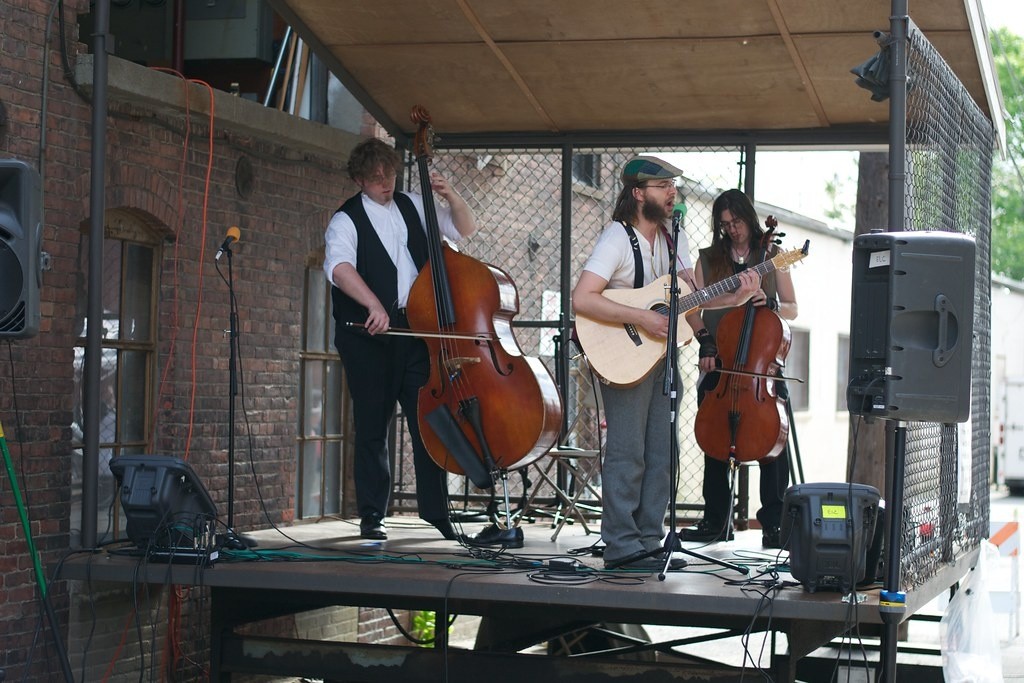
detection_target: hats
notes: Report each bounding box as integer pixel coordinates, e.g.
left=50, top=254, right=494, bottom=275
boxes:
left=620, top=154, right=685, bottom=185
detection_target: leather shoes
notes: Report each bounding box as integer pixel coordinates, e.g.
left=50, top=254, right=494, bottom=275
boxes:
left=461, top=523, right=526, bottom=548
left=679, top=519, right=735, bottom=544
left=361, top=515, right=390, bottom=540
left=762, top=525, right=782, bottom=550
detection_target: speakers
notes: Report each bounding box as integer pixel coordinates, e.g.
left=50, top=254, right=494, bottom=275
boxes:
left=110, top=454, right=217, bottom=549
left=0, top=157, right=41, bottom=341
left=779, top=482, right=883, bottom=594
left=848, top=229, right=976, bottom=426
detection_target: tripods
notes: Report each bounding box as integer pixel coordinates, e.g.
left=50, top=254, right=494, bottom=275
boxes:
left=605, top=223, right=751, bottom=581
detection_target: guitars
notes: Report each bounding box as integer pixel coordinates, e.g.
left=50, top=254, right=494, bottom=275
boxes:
left=573, top=241, right=809, bottom=389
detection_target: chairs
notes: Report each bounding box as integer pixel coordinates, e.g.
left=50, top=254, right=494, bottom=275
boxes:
left=514, top=377, right=609, bottom=541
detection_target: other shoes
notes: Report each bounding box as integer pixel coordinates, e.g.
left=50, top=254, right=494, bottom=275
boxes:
left=645, top=547, right=690, bottom=568
left=602, top=549, right=673, bottom=571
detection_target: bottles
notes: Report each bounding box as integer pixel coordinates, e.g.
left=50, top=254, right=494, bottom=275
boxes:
left=918, top=507, right=936, bottom=560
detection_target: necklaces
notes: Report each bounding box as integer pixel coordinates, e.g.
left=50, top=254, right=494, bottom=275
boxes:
left=732, top=245, right=749, bottom=264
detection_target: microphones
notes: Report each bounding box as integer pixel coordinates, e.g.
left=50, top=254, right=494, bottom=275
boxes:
left=671, top=202, right=687, bottom=221
left=215, top=226, right=241, bottom=261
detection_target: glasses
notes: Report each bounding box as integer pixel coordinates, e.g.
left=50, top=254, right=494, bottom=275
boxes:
left=366, top=168, right=398, bottom=186
left=640, top=178, right=677, bottom=188
left=721, top=218, right=745, bottom=232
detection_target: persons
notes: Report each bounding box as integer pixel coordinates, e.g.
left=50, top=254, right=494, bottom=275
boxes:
left=679, top=189, right=798, bottom=547
left=323, top=138, right=476, bottom=540
left=571, top=155, right=759, bottom=570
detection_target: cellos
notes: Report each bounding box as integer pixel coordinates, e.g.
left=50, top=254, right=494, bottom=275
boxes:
left=695, top=216, right=791, bottom=542
left=406, top=104, right=564, bottom=531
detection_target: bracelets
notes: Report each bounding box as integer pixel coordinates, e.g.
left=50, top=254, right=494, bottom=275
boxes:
left=696, top=328, right=707, bottom=339
left=772, top=298, right=781, bottom=313
